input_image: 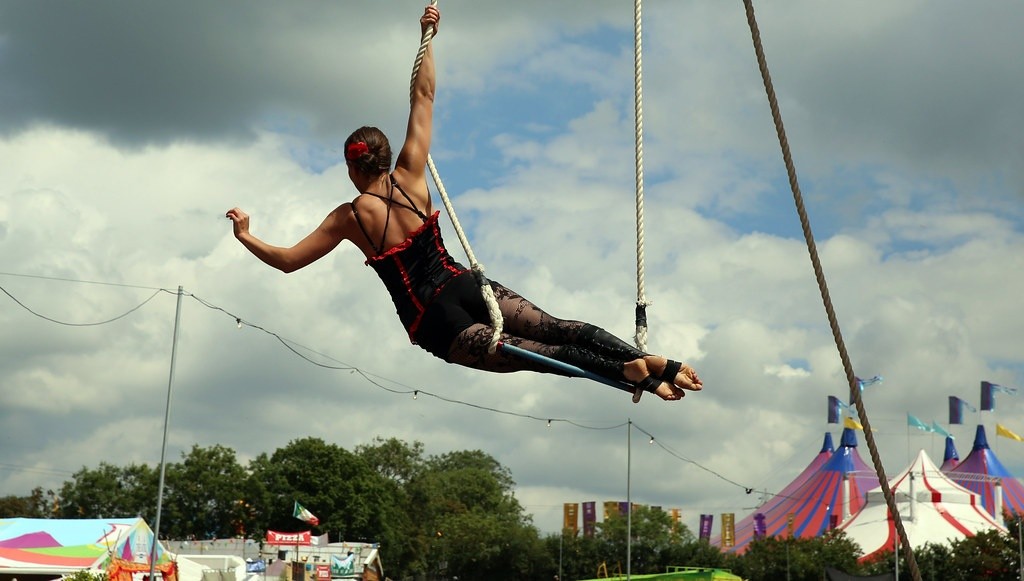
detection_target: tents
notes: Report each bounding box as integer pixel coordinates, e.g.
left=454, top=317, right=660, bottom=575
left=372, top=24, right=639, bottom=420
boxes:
left=0, top=518, right=180, bottom=581
left=708, top=417, right=1024, bottom=575
left=245, top=557, right=265, bottom=573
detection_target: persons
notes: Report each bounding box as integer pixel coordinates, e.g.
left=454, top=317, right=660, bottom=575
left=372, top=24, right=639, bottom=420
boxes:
left=226, top=5, right=702, bottom=402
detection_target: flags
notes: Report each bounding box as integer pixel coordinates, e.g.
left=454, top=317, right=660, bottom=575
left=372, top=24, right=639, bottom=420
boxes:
left=293, top=501, right=318, bottom=526
